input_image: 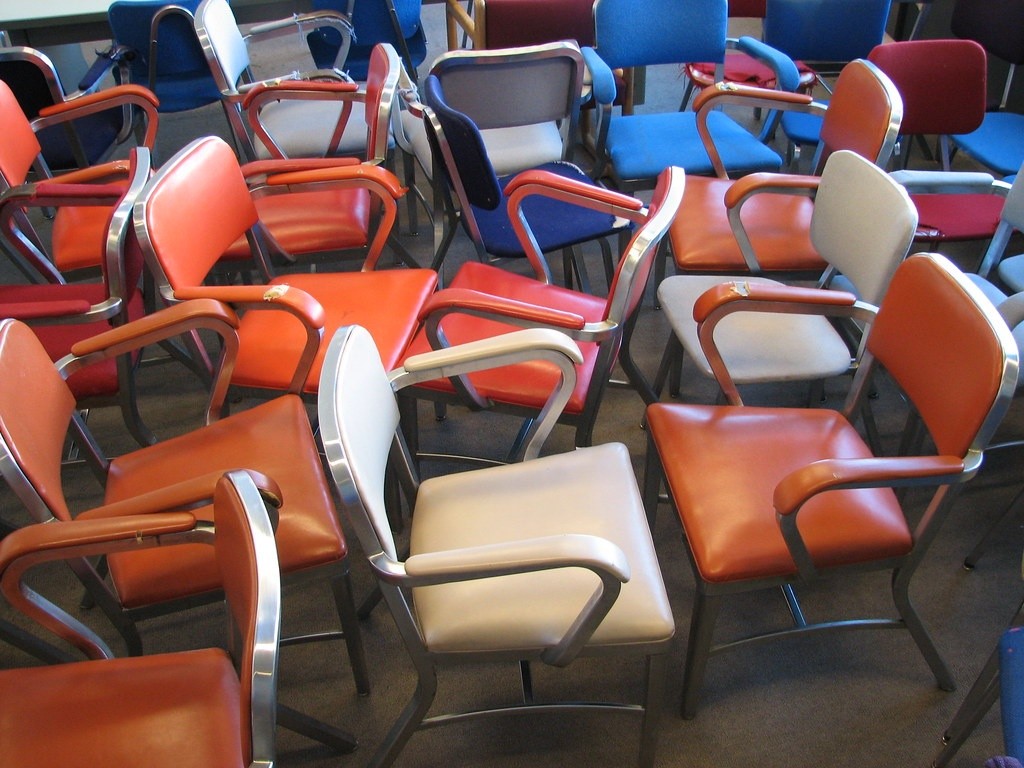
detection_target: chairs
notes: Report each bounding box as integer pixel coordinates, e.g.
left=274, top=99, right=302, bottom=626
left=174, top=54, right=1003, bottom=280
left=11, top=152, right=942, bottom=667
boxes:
left=445, top=0, right=634, bottom=180
left=0, top=299, right=370, bottom=701
left=207, top=43, right=422, bottom=309
left=591, top=0, right=1024, bottom=768
left=0, top=147, right=243, bottom=470
left=0, top=45, right=139, bottom=222
left=0, top=470, right=359, bottom=768
left=0, top=77, right=161, bottom=282
left=393, top=164, right=687, bottom=517
left=308, top=0, right=427, bottom=83
left=423, top=73, right=635, bottom=297
left=107, top=0, right=257, bottom=170
left=391, top=40, right=593, bottom=237
left=317, top=325, right=676, bottom=768
left=132, top=136, right=440, bottom=536
left=193, top=0, right=407, bottom=268
